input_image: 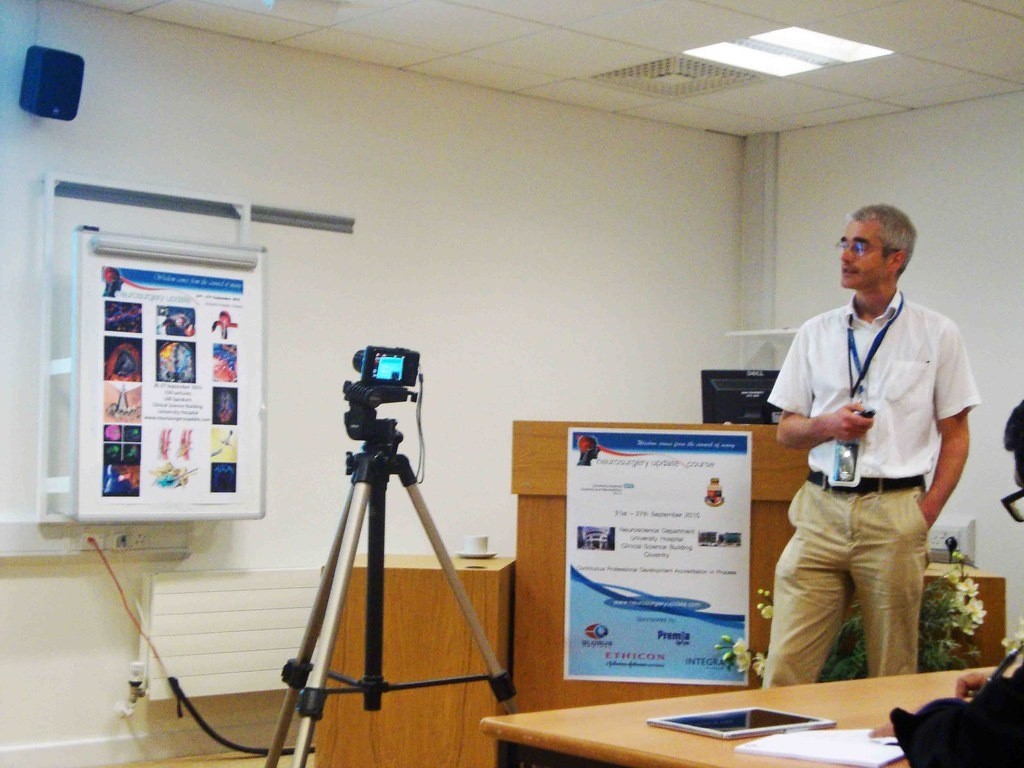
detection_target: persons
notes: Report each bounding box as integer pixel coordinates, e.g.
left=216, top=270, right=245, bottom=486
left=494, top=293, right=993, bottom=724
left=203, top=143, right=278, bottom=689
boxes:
left=762, top=204, right=981, bottom=688
left=869, top=400, right=1024, bottom=767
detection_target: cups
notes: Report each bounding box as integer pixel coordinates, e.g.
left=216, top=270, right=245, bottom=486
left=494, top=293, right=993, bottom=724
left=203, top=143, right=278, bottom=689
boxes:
left=464, top=535, right=487, bottom=553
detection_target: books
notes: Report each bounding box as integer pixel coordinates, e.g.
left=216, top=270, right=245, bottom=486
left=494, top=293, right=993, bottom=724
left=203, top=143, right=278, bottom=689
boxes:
left=735, top=727, right=905, bottom=767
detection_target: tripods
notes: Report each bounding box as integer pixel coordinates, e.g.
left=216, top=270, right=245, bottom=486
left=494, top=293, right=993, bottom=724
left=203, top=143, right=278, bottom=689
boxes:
left=263, top=378, right=517, bottom=768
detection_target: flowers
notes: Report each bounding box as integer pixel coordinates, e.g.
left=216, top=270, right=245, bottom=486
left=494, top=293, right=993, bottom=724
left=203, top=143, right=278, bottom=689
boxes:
left=713, top=551, right=1024, bottom=686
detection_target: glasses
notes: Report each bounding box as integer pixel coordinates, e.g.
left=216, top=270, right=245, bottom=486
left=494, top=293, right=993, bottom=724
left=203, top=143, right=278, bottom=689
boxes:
left=836, top=239, right=886, bottom=258
left=1000, top=485, right=1024, bottom=522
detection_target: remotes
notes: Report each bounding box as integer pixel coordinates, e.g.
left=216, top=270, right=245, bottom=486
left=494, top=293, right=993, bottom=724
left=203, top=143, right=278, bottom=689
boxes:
left=861, top=408, right=877, bottom=419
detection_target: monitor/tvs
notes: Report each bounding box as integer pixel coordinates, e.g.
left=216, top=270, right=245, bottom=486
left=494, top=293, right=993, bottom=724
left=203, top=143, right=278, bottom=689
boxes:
left=699, top=368, right=785, bottom=425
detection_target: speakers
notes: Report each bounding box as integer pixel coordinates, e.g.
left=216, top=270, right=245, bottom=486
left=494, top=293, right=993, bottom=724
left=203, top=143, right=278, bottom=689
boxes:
left=19, top=44, right=84, bottom=120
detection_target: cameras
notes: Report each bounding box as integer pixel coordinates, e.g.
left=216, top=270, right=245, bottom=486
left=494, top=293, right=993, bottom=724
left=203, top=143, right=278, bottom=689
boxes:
left=352, top=345, right=420, bottom=386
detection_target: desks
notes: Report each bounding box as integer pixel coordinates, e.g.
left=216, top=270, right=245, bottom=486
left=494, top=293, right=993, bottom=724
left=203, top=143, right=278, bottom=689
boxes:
left=482, top=665, right=1024, bottom=768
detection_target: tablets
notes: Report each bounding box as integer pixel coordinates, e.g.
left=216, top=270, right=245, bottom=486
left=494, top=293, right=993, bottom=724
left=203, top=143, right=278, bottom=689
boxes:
left=646, top=707, right=836, bottom=742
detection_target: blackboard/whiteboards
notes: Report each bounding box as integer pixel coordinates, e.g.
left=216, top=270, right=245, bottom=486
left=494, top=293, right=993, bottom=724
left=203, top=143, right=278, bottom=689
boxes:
left=73, top=225, right=268, bottom=525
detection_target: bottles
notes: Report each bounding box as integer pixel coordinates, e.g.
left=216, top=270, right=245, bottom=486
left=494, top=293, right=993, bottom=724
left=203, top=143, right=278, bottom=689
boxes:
left=707, top=478, right=722, bottom=504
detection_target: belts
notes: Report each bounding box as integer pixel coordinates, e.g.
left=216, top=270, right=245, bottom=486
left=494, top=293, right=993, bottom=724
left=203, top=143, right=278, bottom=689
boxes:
left=805, top=471, right=924, bottom=495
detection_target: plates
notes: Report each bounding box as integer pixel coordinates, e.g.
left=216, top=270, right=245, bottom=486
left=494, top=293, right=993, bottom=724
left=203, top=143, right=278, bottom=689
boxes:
left=455, top=551, right=497, bottom=558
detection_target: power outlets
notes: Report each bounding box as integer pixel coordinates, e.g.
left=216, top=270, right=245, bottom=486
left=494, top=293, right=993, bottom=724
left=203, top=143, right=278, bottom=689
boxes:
left=928, top=517, right=976, bottom=565
